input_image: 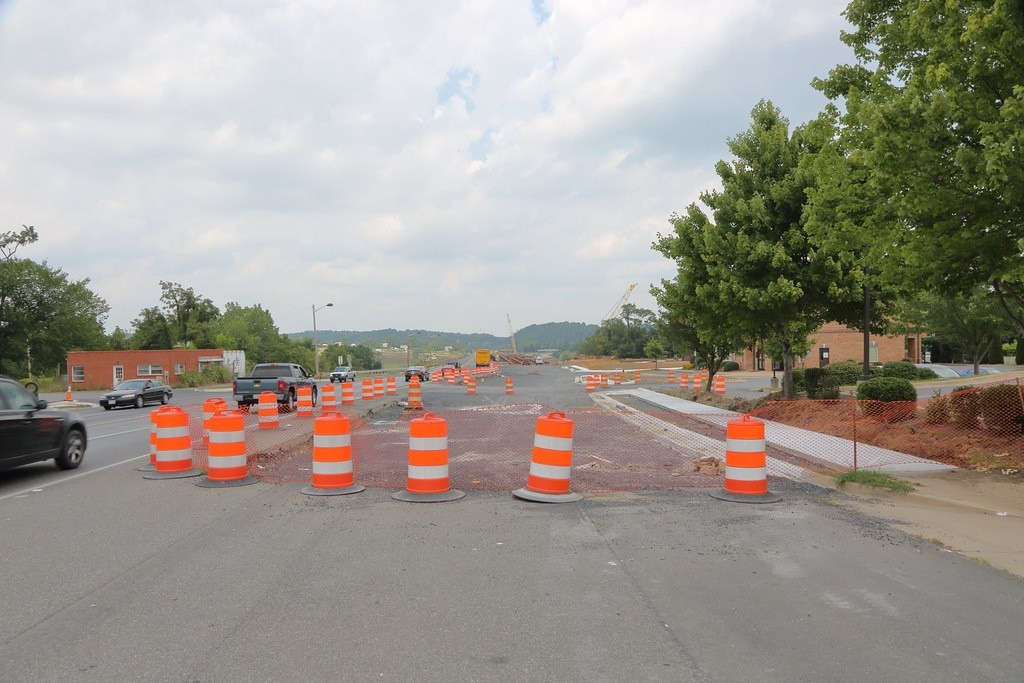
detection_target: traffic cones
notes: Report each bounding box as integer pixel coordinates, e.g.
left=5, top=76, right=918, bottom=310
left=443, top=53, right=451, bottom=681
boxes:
left=64, top=383, right=73, bottom=401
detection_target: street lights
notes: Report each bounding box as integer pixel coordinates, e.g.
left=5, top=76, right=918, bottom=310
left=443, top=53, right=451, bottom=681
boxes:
left=407, top=332, right=420, bottom=370
left=312, top=303, right=333, bottom=378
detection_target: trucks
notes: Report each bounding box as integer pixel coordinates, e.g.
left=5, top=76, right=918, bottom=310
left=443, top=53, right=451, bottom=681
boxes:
left=475, top=349, right=490, bottom=367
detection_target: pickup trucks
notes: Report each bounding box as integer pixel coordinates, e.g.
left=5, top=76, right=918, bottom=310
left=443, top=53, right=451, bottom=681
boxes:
left=329, top=366, right=356, bottom=383
left=232, top=363, right=318, bottom=413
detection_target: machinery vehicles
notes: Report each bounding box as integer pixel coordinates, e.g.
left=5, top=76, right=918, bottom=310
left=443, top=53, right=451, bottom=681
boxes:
left=506, top=315, right=517, bottom=354
left=600, top=281, right=639, bottom=327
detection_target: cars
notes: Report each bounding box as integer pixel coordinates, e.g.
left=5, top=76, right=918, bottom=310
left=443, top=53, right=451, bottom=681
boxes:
left=0, top=375, right=87, bottom=474
left=99, top=379, right=174, bottom=410
left=440, top=361, right=462, bottom=374
left=536, top=357, right=544, bottom=365
left=405, top=366, right=430, bottom=382
